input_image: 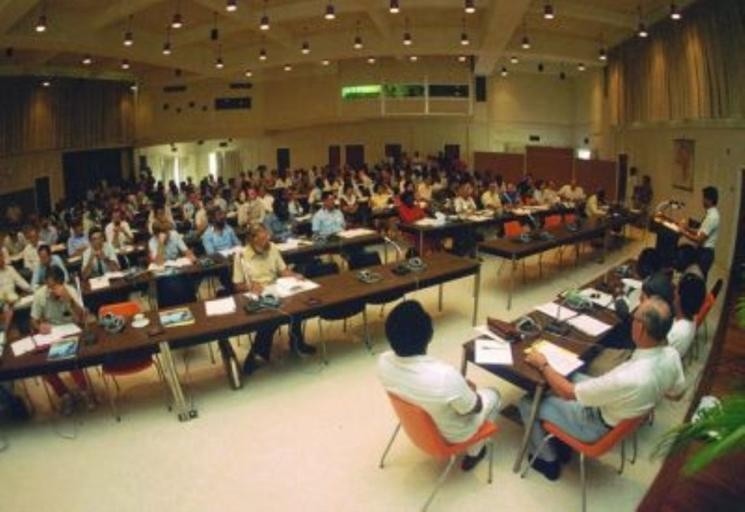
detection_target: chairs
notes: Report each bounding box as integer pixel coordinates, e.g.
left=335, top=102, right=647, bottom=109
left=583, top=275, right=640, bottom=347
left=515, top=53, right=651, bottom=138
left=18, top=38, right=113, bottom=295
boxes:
left=379, top=215, right=722, bottom=512
left=379, top=197, right=648, bottom=314
left=0, top=204, right=377, bottom=423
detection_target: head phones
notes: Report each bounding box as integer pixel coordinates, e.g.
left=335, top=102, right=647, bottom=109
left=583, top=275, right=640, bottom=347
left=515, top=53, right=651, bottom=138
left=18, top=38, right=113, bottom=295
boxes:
left=517, top=317, right=543, bottom=336
left=263, top=292, right=279, bottom=307
left=104, top=312, right=125, bottom=333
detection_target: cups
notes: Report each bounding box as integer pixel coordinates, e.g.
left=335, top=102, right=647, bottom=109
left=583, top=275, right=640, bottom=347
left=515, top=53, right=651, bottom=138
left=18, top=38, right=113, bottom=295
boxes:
left=134, top=314, right=144, bottom=324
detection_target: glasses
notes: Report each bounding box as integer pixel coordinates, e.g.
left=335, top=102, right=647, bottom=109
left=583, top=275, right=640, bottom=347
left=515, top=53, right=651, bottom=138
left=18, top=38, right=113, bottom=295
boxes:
left=627, top=312, right=641, bottom=323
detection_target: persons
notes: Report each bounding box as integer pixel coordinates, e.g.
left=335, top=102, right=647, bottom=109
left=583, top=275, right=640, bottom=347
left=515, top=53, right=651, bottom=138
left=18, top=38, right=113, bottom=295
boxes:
left=638, top=185, right=721, bottom=368
left=376, top=298, right=500, bottom=470
left=2, top=150, right=653, bottom=407
left=515, top=295, right=688, bottom=481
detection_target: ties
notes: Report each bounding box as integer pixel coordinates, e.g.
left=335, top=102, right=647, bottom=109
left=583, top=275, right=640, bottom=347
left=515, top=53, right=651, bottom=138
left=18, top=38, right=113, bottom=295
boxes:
left=96, top=254, right=102, bottom=275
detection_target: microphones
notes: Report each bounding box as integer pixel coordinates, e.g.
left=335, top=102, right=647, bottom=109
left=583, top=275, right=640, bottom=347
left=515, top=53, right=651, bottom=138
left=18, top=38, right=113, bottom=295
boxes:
left=521, top=207, right=543, bottom=239
left=545, top=292, right=601, bottom=337
left=670, top=200, right=687, bottom=208
left=381, top=231, right=410, bottom=275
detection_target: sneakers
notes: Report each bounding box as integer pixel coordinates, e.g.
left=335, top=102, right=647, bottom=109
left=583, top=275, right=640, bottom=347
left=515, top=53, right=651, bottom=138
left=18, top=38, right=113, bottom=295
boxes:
left=82, top=394, right=97, bottom=412
left=64, top=396, right=77, bottom=416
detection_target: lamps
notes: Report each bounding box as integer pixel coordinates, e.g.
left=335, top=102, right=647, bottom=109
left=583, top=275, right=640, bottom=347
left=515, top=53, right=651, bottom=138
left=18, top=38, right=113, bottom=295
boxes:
left=33, top=0, right=683, bottom=80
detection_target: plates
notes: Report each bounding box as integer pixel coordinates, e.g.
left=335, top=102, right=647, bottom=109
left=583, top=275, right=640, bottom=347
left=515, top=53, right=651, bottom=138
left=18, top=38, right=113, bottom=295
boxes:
left=131, top=319, right=151, bottom=328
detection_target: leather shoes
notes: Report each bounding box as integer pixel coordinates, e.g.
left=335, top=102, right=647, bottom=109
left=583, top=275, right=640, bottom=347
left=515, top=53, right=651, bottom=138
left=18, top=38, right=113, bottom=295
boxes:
left=244, top=358, right=252, bottom=375
left=462, top=444, right=485, bottom=470
left=556, top=443, right=570, bottom=461
left=298, top=342, right=317, bottom=355
left=528, top=454, right=559, bottom=480
